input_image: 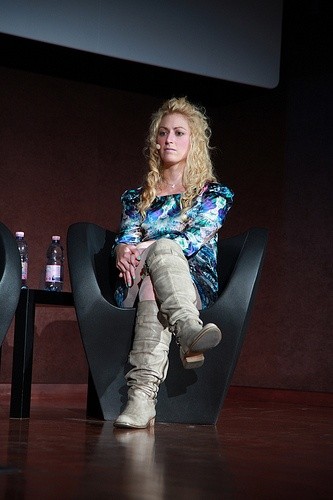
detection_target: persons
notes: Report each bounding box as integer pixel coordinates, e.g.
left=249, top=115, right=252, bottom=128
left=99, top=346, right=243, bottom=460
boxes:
left=112, top=96, right=234, bottom=428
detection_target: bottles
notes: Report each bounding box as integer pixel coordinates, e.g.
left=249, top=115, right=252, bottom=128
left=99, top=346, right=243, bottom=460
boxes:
left=15, top=231, right=28, bottom=289
left=45, top=235, right=65, bottom=292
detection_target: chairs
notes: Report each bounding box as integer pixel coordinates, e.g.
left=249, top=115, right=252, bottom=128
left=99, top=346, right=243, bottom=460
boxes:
left=66, top=220, right=270, bottom=425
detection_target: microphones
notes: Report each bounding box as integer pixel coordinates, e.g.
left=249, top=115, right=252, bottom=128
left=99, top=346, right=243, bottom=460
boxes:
left=156, top=143, right=161, bottom=150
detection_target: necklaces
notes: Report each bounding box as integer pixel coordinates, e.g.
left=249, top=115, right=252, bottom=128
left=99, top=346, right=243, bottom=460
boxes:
left=161, top=178, right=183, bottom=188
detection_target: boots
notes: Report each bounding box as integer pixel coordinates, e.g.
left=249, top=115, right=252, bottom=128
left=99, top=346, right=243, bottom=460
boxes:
left=146, top=236, right=222, bottom=370
left=112, top=299, right=172, bottom=430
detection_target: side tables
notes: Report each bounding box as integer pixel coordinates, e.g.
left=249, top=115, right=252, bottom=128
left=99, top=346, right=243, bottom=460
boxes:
left=9, top=287, right=104, bottom=421
